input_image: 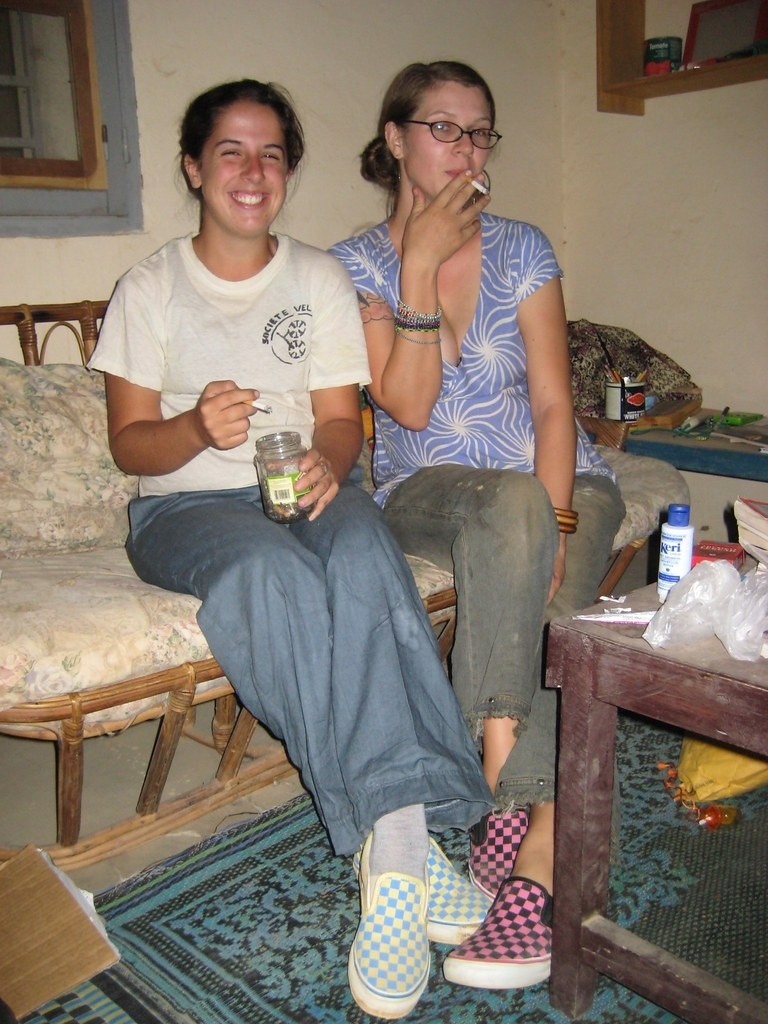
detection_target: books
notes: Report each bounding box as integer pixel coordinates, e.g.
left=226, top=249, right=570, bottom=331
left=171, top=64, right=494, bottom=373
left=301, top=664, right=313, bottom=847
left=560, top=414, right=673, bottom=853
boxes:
left=690, top=417, right=768, bottom=581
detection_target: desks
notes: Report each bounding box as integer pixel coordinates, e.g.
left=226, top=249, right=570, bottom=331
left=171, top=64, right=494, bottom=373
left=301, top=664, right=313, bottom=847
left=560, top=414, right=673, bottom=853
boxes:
left=586, top=408, right=768, bottom=584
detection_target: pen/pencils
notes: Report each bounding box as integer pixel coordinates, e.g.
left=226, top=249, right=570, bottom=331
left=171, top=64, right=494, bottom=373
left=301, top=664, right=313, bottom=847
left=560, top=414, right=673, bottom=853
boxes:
left=600, top=365, right=648, bottom=383
left=713, top=407, right=730, bottom=431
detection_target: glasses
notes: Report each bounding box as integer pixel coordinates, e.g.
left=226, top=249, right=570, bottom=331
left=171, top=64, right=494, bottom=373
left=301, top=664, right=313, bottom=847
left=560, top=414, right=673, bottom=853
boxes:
left=402, top=120, right=502, bottom=149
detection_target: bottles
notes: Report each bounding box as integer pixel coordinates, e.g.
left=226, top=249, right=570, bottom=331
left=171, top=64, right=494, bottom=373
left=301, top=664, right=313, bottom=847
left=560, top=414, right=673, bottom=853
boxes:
left=657, top=504, right=693, bottom=606
left=252, top=430, right=317, bottom=523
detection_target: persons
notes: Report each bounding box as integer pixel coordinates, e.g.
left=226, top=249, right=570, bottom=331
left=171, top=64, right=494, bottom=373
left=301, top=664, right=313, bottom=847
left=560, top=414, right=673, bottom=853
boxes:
left=327, top=60, right=624, bottom=990
left=88, top=80, right=493, bottom=1018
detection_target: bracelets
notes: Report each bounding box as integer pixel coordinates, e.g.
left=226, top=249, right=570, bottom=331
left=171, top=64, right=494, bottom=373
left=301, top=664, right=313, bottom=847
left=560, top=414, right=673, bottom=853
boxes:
left=392, top=300, right=443, bottom=345
left=553, top=508, right=580, bottom=535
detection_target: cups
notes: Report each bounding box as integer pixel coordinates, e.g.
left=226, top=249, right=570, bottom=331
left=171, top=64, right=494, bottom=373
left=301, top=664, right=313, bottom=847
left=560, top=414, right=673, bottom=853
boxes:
left=605, top=381, right=646, bottom=420
left=642, top=36, right=683, bottom=77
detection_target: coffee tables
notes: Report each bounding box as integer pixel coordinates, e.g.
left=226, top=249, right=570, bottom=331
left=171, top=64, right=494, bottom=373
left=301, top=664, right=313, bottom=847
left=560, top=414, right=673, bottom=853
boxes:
left=545, top=557, right=768, bottom=1024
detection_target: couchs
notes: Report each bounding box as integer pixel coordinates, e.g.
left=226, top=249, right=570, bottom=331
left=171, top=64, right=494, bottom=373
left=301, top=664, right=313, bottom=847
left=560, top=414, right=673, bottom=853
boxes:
left=0, top=299, right=692, bottom=873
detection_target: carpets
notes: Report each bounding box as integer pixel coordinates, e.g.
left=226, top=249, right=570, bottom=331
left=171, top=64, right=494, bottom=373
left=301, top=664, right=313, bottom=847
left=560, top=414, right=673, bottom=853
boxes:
left=0, top=713, right=768, bottom=1024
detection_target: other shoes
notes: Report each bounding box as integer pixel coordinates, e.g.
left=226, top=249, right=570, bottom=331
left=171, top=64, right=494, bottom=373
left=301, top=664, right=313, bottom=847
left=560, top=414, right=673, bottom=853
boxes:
left=346, top=832, right=431, bottom=1019
left=443, top=876, right=553, bottom=990
left=353, top=837, right=492, bottom=944
left=468, top=808, right=529, bottom=901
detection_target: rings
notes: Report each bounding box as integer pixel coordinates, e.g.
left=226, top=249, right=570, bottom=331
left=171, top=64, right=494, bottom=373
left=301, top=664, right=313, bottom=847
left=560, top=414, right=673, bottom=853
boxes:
left=314, top=461, right=329, bottom=476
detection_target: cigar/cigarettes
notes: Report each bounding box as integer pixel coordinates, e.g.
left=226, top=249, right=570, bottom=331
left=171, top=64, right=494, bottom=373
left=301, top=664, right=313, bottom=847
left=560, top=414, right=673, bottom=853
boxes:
left=467, top=178, right=490, bottom=196
left=242, top=400, right=273, bottom=414
